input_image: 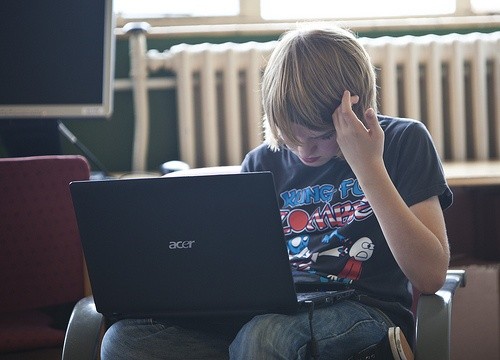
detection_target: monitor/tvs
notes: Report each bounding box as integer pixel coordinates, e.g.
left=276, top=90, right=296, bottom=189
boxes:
left=0, top=0, right=114, bottom=117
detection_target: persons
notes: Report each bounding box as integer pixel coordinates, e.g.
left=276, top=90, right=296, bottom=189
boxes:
left=100, top=28, right=452, bottom=360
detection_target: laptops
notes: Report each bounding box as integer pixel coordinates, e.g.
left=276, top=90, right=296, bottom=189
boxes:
left=72, top=171, right=355, bottom=320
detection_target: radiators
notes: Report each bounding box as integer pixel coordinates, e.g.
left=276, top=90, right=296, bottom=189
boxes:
left=126, top=23, right=499, bottom=167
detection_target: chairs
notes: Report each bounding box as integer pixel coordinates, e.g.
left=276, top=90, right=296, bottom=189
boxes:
left=61, top=160, right=468, bottom=359
left=1, top=156, right=88, bottom=355
